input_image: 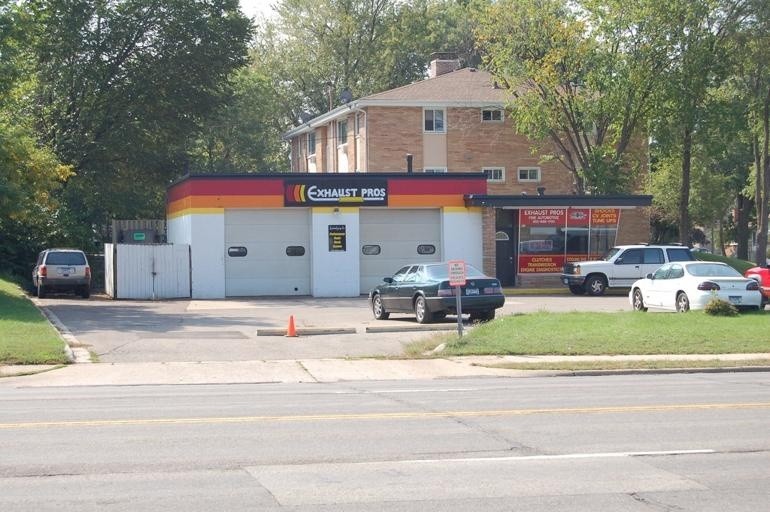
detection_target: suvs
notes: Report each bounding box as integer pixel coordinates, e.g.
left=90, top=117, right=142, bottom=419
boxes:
left=30, top=246, right=93, bottom=297
left=559, top=240, right=702, bottom=300
left=743, top=262, right=770, bottom=310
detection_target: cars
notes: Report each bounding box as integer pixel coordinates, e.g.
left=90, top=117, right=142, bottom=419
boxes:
left=542, top=228, right=616, bottom=255
left=690, top=246, right=712, bottom=254
left=367, top=262, right=508, bottom=325
left=629, top=259, right=763, bottom=312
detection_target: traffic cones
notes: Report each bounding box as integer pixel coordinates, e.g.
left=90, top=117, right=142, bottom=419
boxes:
left=285, top=314, right=300, bottom=338
